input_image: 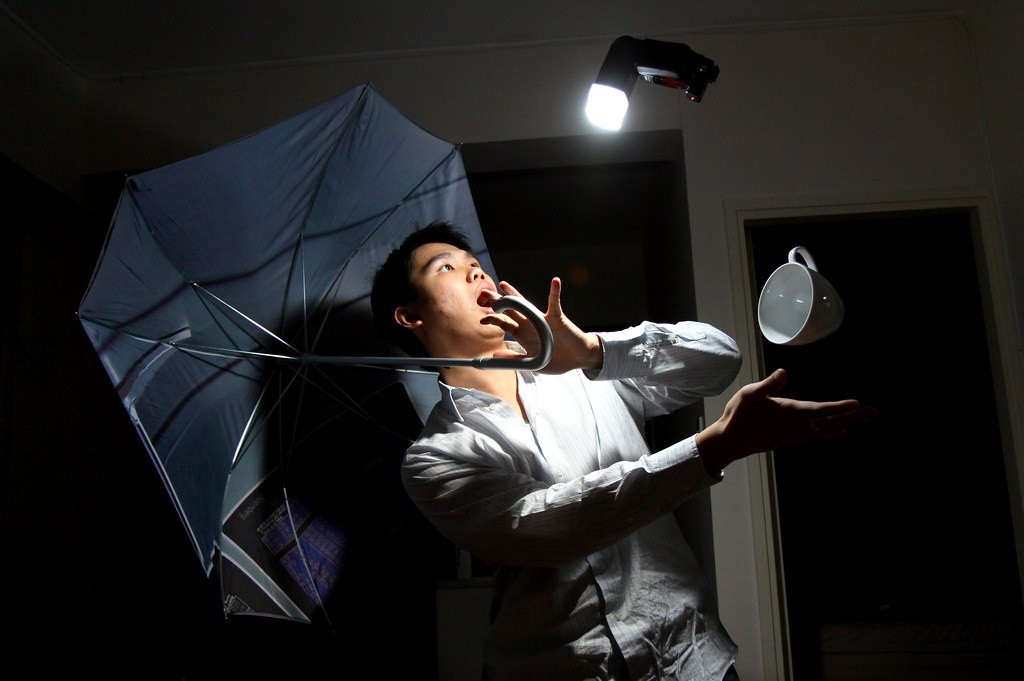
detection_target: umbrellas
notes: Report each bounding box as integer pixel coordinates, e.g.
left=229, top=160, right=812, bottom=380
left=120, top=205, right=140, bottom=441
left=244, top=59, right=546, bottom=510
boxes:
left=76, top=80, right=554, bottom=627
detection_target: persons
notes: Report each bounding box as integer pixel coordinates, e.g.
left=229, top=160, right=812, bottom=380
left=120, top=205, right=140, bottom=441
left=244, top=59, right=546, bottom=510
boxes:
left=371, top=222, right=860, bottom=681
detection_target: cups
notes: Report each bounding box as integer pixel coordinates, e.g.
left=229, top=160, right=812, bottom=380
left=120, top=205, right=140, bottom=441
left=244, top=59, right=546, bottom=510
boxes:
left=758, top=246, right=844, bottom=346
